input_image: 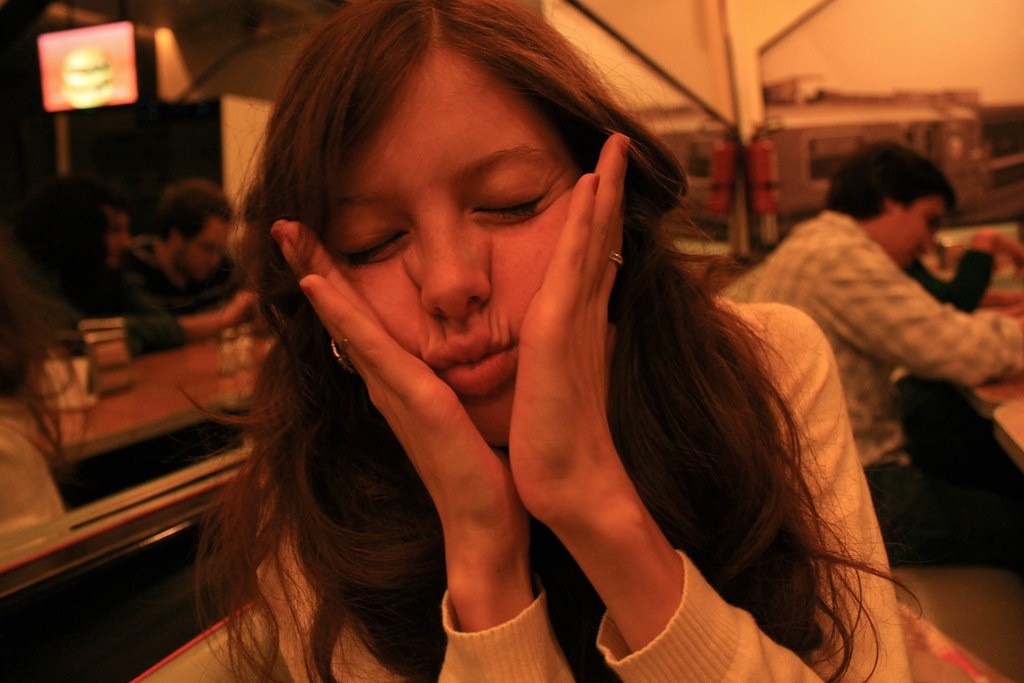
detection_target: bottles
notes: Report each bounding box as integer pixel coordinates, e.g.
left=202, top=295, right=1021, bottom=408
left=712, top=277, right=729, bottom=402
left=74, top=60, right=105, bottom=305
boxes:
left=218, top=323, right=257, bottom=404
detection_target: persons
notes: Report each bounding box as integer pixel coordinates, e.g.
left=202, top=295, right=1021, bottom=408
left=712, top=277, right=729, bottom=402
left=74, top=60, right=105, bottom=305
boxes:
left=716, top=138, right=1024, bottom=572
left=0, top=227, right=75, bottom=534
left=44, top=169, right=236, bottom=350
left=199, top=0, right=908, bottom=683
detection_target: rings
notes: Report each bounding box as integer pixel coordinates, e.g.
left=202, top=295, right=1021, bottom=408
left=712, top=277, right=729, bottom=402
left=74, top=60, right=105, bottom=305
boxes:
left=331, top=339, right=354, bottom=374
left=609, top=251, right=624, bottom=265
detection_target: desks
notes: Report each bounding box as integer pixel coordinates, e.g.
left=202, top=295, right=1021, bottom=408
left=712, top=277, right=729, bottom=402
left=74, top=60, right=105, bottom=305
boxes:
left=920, top=223, right=1024, bottom=469
left=45, top=339, right=273, bottom=468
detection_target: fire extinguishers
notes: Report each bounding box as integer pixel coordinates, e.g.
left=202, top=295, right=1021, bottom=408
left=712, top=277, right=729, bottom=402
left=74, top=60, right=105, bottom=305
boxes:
left=746, top=115, right=785, bottom=212
left=697, top=125, right=738, bottom=213
